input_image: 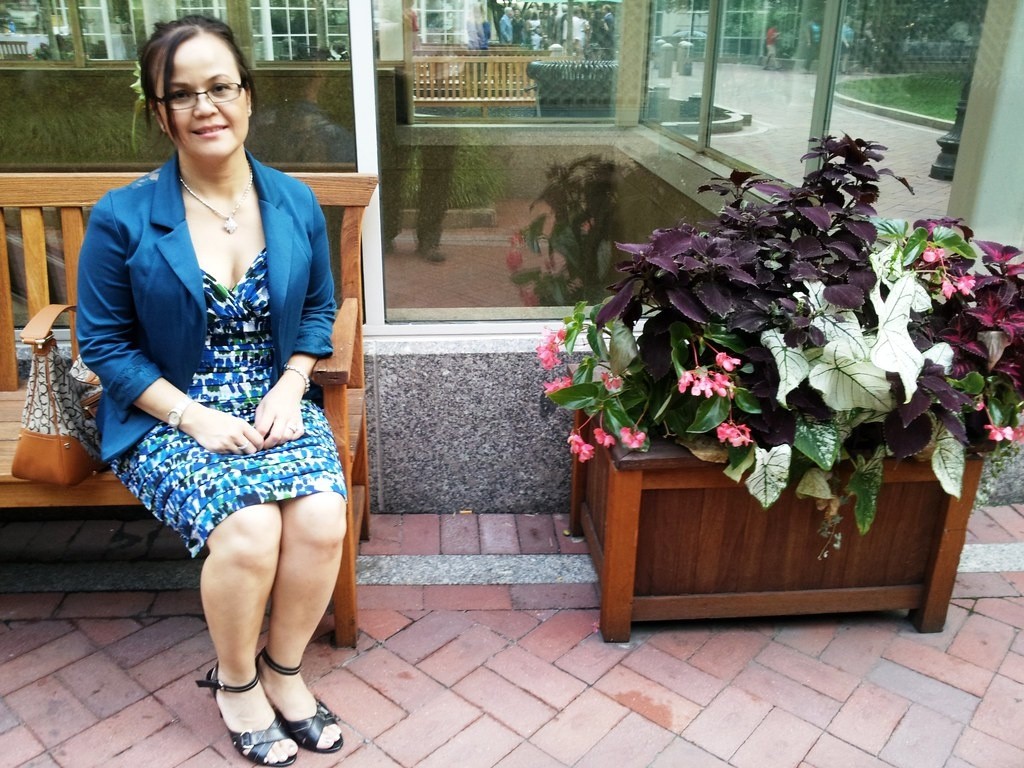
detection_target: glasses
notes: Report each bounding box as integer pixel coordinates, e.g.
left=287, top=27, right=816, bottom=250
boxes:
left=153, top=79, right=244, bottom=111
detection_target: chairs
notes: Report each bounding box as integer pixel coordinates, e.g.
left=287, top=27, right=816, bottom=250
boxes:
left=1, top=40, right=29, bottom=61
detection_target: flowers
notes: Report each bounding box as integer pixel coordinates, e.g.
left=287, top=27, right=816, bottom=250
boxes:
left=539, top=131, right=1023, bottom=561
left=506, top=155, right=620, bottom=308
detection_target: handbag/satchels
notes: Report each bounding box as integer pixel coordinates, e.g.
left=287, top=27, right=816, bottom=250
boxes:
left=11, top=304, right=110, bottom=488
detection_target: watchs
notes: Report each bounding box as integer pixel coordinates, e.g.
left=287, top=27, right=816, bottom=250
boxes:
left=168, top=397, right=189, bottom=429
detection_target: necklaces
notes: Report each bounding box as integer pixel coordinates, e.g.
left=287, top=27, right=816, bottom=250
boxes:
left=181, top=160, right=254, bottom=234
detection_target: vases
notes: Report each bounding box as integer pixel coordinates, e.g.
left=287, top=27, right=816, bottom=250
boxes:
left=565, top=394, right=984, bottom=642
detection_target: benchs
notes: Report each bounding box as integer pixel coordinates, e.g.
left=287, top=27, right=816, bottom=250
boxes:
left=0, top=170, right=376, bottom=650
left=412, top=44, right=577, bottom=117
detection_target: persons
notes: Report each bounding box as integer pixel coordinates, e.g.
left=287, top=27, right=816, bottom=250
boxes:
left=762, top=19, right=783, bottom=71
left=488, top=1, right=617, bottom=58
left=802, top=15, right=945, bottom=74
left=350, top=0, right=485, bottom=262
left=946, top=10, right=972, bottom=68
left=77, top=14, right=348, bottom=766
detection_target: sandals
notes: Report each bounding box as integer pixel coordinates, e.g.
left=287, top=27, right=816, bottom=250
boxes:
left=256, top=646, right=344, bottom=753
left=195, top=659, right=298, bottom=768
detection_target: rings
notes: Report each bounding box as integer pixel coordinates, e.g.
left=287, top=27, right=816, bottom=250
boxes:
left=239, top=441, right=251, bottom=450
left=288, top=423, right=298, bottom=432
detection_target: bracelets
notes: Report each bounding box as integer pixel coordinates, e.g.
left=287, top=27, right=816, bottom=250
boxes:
left=285, top=365, right=310, bottom=393
